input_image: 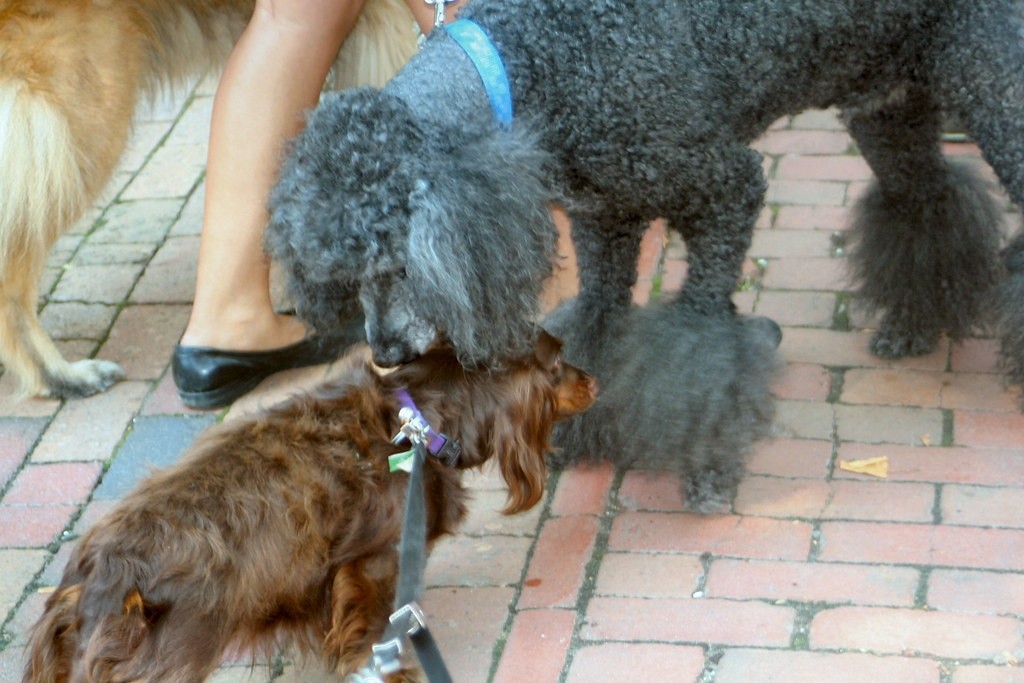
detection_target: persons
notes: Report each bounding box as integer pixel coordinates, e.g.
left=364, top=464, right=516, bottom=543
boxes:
left=173, top=0, right=471, bottom=408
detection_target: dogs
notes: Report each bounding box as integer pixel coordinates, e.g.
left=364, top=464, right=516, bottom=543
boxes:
left=21, top=321, right=601, bottom=682
left=254, top=0, right=1024, bottom=516
left=1, top=0, right=428, bottom=403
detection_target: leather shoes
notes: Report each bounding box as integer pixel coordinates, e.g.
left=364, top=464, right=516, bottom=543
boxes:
left=170, top=310, right=346, bottom=409
